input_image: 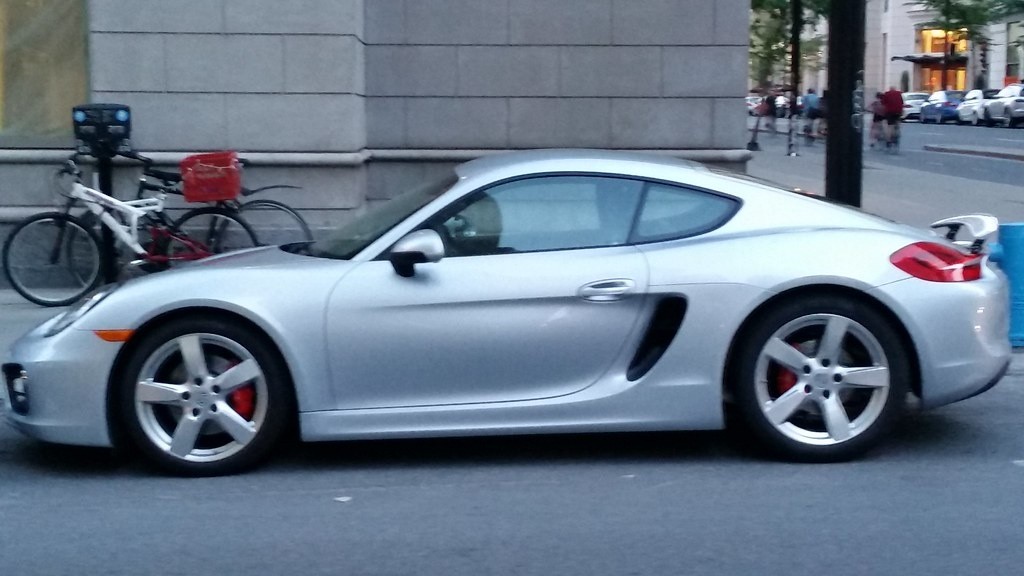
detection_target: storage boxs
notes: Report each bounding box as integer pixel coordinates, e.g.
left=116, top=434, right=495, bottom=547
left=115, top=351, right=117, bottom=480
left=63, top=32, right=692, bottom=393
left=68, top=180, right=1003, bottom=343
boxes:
left=179, top=151, right=241, bottom=202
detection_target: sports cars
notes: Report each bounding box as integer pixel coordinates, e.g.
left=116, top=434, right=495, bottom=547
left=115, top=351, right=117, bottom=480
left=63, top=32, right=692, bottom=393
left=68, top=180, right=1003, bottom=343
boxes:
left=0, top=148, right=1017, bottom=479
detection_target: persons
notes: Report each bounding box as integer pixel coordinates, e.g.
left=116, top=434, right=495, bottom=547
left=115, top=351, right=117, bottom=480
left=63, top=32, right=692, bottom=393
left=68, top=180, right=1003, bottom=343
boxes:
left=754, top=88, right=827, bottom=119
left=901, top=70, right=909, bottom=92
left=870, top=85, right=904, bottom=147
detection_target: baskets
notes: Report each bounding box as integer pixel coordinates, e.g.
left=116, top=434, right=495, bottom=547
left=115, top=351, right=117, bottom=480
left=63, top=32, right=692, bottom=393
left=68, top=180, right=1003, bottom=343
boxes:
left=180, top=151, right=237, bottom=202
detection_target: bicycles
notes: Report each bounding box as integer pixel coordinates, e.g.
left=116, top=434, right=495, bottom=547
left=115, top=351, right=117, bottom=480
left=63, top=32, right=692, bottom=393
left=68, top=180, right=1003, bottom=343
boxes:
left=3, top=144, right=315, bottom=311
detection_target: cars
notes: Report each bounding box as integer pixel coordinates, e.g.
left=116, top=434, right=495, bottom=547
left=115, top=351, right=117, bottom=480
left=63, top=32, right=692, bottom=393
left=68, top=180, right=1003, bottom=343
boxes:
left=955, top=89, right=1003, bottom=126
left=902, top=91, right=932, bottom=122
left=982, top=83, right=1024, bottom=129
left=745, top=96, right=762, bottom=115
left=918, top=90, right=969, bottom=125
left=764, top=95, right=805, bottom=109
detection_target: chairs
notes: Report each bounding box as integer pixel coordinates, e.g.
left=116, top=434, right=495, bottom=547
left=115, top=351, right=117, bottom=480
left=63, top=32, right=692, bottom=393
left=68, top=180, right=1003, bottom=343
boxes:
left=594, top=180, right=642, bottom=244
